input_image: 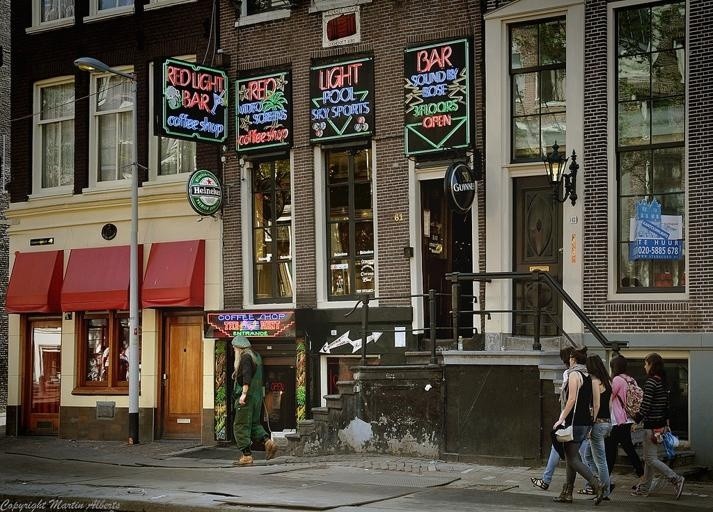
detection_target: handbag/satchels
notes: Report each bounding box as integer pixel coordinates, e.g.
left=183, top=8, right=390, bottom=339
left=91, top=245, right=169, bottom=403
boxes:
left=554, top=426, right=574, bottom=442
left=652, top=428, right=671, bottom=444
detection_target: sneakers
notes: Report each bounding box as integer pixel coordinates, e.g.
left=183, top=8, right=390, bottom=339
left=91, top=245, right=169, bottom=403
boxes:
left=233, top=455, right=253, bottom=465
left=531, top=476, right=685, bottom=505
left=265, top=440, right=276, bottom=460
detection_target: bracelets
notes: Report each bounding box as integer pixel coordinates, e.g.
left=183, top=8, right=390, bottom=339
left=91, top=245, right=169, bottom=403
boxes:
left=242, top=391, right=247, bottom=397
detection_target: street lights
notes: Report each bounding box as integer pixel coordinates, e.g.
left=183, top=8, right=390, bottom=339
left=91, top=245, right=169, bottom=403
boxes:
left=70, top=57, right=143, bottom=443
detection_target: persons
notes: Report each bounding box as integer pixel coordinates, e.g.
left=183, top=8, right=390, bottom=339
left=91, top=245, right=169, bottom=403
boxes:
left=586, top=355, right=613, bottom=502
left=531, top=346, right=595, bottom=496
left=604, top=355, right=644, bottom=483
left=232, top=335, right=279, bottom=465
left=553, top=346, right=608, bottom=505
left=631, top=353, right=686, bottom=500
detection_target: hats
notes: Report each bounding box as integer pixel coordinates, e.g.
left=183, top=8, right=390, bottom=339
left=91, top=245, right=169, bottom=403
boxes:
left=232, top=336, right=251, bottom=348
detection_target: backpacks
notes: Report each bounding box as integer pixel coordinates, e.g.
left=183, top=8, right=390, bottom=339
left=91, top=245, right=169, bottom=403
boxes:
left=625, top=380, right=644, bottom=419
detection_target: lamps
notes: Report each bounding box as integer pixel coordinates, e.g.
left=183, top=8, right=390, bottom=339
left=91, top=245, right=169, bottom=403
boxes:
left=542, top=140, right=579, bottom=207
